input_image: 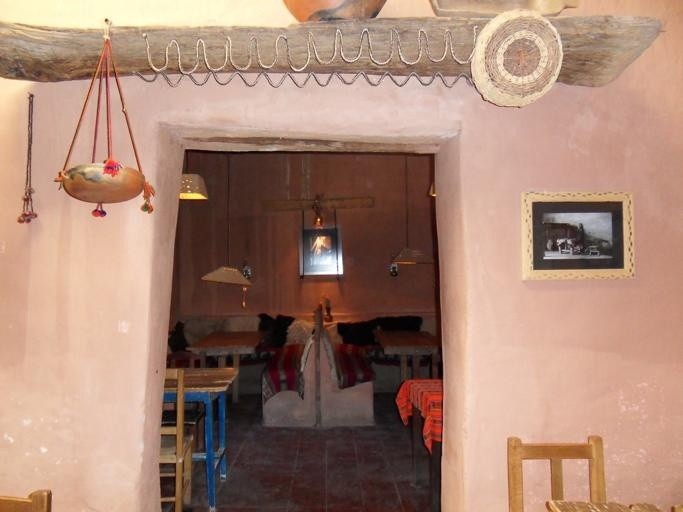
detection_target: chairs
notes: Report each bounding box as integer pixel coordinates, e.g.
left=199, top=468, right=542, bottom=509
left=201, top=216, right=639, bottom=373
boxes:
left=161, top=348, right=204, bottom=511
left=504, top=435, right=607, bottom=512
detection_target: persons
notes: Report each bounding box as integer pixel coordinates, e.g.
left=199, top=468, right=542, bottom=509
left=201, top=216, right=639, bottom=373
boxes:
left=577, top=223, right=585, bottom=254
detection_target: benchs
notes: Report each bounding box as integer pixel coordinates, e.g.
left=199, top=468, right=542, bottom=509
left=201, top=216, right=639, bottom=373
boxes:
left=261, top=295, right=376, bottom=429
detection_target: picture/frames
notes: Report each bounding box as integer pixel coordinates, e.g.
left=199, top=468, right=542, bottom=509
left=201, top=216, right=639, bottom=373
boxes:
left=300, top=223, right=345, bottom=277
left=517, top=189, right=639, bottom=285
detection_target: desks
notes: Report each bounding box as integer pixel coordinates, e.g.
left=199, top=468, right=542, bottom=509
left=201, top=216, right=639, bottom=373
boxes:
left=185, top=331, right=264, bottom=404
left=161, top=366, right=240, bottom=512
left=372, top=327, right=440, bottom=512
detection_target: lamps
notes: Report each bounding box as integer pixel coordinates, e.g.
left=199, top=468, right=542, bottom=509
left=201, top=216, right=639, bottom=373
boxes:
left=178, top=150, right=250, bottom=288
left=393, top=153, right=438, bottom=266
